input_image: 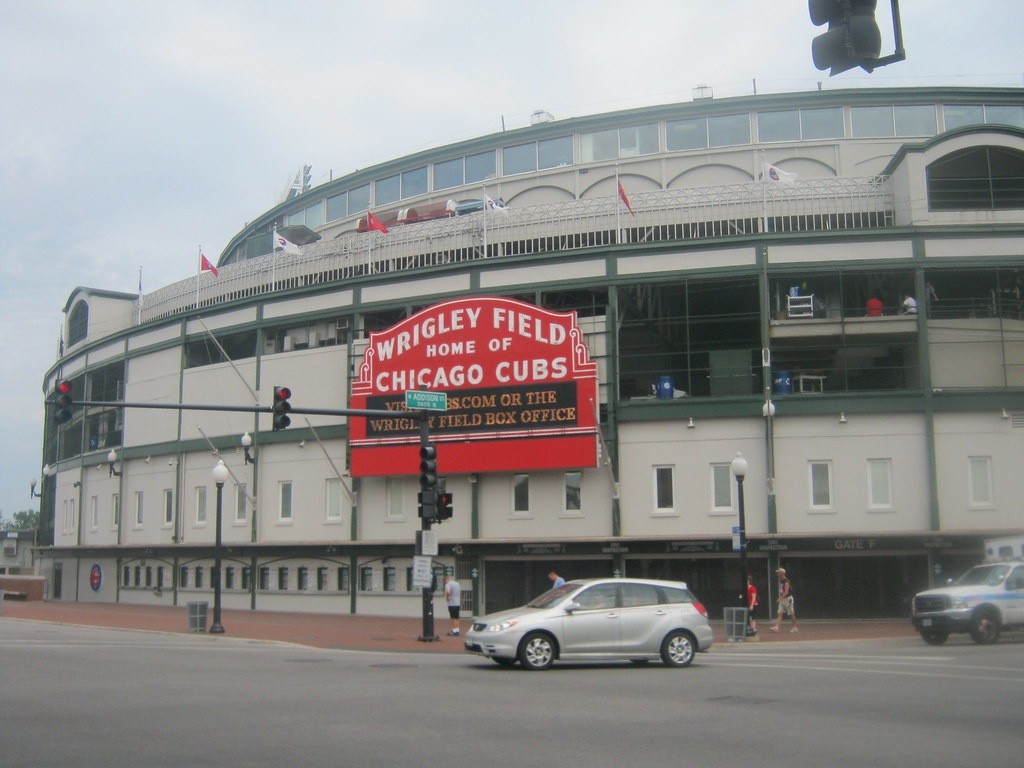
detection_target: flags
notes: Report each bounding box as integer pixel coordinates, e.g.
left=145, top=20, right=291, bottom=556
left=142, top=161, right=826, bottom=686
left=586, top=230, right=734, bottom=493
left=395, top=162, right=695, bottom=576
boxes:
left=618, top=180, right=636, bottom=217
left=200, top=252, right=218, bottom=279
left=367, top=211, right=388, bottom=234
left=761, top=160, right=797, bottom=184
left=486, top=194, right=504, bottom=214
left=273, top=231, right=303, bottom=255
left=139, top=271, right=142, bottom=305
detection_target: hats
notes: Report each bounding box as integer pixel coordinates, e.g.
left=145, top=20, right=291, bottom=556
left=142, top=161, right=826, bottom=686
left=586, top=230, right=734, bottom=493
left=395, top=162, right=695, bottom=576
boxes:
left=775, top=568, right=786, bottom=573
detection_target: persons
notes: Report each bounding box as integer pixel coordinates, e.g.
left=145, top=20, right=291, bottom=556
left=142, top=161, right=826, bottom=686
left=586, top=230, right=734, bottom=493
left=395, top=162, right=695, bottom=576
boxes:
left=577, top=591, right=607, bottom=610
left=442, top=577, right=461, bottom=637
left=740, top=576, right=758, bottom=634
left=864, top=294, right=883, bottom=316
left=548, top=571, right=565, bottom=587
left=768, top=568, right=800, bottom=633
left=925, top=279, right=938, bottom=318
left=901, top=290, right=917, bottom=316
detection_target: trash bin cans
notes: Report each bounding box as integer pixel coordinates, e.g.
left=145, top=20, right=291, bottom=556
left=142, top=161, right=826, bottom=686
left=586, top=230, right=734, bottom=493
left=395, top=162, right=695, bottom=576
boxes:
left=659, top=376, right=674, bottom=399
left=187, top=601, right=208, bottom=632
left=724, top=606, right=748, bottom=643
left=774, top=371, right=794, bottom=395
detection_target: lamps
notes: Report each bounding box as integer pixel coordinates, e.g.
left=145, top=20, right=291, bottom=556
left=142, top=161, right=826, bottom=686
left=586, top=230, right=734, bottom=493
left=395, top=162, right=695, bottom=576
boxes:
left=242, top=432, right=255, bottom=465
left=107, top=448, right=120, bottom=478
left=43, top=464, right=54, bottom=490
left=29, top=479, right=40, bottom=499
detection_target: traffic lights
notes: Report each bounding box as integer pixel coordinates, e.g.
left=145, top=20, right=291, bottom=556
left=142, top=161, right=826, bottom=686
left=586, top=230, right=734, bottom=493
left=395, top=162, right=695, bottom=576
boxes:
left=55, top=378, right=72, bottom=423
left=807, top=0, right=859, bottom=78
left=273, top=386, right=291, bottom=431
left=438, top=493, right=453, bottom=521
left=418, top=443, right=438, bottom=491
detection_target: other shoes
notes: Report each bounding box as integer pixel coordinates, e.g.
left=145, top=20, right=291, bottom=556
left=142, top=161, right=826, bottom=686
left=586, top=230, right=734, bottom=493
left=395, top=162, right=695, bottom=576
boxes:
left=447, top=630, right=459, bottom=637
left=769, top=626, right=779, bottom=632
left=790, top=626, right=798, bottom=632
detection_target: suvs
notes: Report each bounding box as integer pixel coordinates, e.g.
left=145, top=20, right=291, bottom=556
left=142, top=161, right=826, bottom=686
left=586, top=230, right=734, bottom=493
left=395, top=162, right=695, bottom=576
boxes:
left=465, top=576, right=714, bottom=670
left=908, top=560, right=1024, bottom=645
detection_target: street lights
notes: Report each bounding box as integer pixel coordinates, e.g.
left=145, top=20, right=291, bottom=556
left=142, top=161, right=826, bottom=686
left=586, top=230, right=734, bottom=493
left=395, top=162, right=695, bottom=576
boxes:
left=209, top=459, right=228, bottom=634
left=730, top=450, right=749, bottom=606
